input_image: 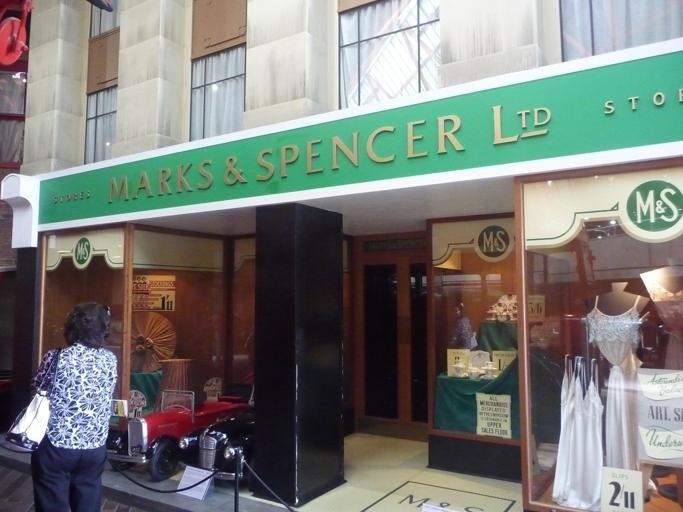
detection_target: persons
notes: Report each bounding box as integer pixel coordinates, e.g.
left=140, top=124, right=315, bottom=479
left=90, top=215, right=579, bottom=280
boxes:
left=30, top=304, right=116, bottom=511
left=583, top=277, right=652, bottom=501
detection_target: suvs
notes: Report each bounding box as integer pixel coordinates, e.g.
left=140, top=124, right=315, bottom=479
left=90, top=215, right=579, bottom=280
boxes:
left=105, top=390, right=251, bottom=480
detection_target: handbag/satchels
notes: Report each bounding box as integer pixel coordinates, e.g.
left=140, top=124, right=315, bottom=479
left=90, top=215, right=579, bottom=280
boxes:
left=1, top=388, right=54, bottom=453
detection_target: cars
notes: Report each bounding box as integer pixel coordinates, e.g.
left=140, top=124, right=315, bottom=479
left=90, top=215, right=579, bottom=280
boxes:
left=177, top=406, right=252, bottom=480
